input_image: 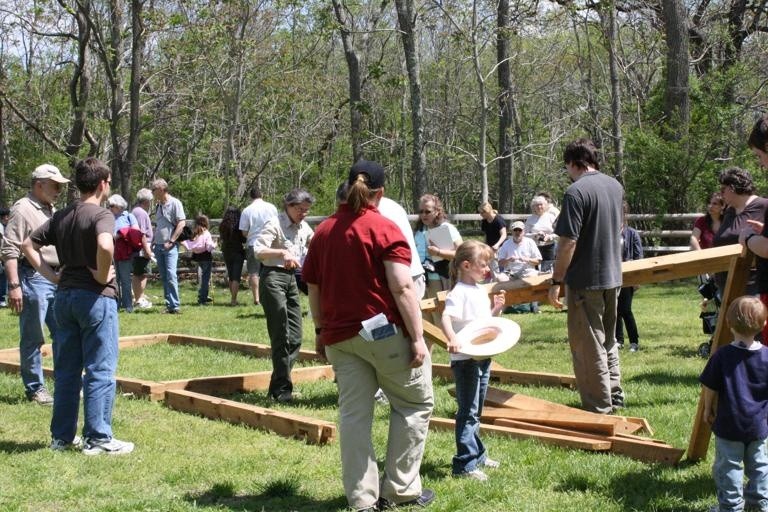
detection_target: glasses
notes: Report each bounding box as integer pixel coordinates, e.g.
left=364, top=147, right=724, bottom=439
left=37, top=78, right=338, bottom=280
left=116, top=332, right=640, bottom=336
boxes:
left=718, top=184, right=729, bottom=192
left=513, top=228, right=523, bottom=232
left=291, top=205, right=310, bottom=216
left=418, top=209, right=438, bottom=215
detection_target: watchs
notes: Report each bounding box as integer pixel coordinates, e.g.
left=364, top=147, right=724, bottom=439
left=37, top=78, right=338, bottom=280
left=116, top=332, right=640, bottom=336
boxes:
left=9, top=281, right=20, bottom=290
left=745, top=233, right=760, bottom=256
left=550, top=279, right=562, bottom=286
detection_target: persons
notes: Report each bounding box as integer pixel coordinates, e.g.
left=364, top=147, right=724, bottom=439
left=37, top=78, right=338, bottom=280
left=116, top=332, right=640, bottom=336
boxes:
left=107, top=195, right=141, bottom=315
left=338, top=184, right=426, bottom=304
left=238, top=188, right=279, bottom=304
left=478, top=202, right=507, bottom=270
left=1, top=164, right=70, bottom=405
left=714, top=167, right=766, bottom=300
left=130, top=188, right=154, bottom=309
left=301, top=160, right=435, bottom=512
left=700, top=296, right=768, bottom=512
left=739, top=117, right=768, bottom=297
left=20, top=157, right=133, bottom=456
left=182, top=215, right=216, bottom=305
left=617, top=219, right=644, bottom=351
left=525, top=196, right=557, bottom=272
left=217, top=204, right=242, bottom=305
left=497, top=222, right=543, bottom=314
left=690, top=194, right=722, bottom=251
left=253, top=191, right=314, bottom=400
left=548, top=138, right=624, bottom=413
left=413, top=192, right=462, bottom=295
left=440, top=239, right=505, bottom=481
left=0, top=209, right=10, bottom=308
left=151, top=178, right=186, bottom=315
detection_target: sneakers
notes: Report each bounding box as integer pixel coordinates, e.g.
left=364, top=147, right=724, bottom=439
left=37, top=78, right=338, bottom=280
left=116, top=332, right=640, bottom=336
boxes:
left=26, top=389, right=54, bottom=407
left=170, top=309, right=184, bottom=315
left=451, top=469, right=489, bottom=483
left=80, top=435, right=135, bottom=456
left=51, top=437, right=84, bottom=451
left=277, top=392, right=293, bottom=402
left=485, top=456, right=500, bottom=468
left=134, top=299, right=152, bottom=309
left=267, top=390, right=302, bottom=403
left=161, top=309, right=170, bottom=314
left=377, top=488, right=435, bottom=512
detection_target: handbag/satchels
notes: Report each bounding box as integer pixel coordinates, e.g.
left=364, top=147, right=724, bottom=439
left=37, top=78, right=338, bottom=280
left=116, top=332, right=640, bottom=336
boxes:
left=158, top=204, right=193, bottom=243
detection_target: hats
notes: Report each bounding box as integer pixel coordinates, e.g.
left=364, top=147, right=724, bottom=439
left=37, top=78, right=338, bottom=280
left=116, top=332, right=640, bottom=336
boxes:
left=450, top=316, right=522, bottom=362
left=509, top=220, right=525, bottom=230
left=347, top=160, right=387, bottom=189
left=30, top=163, right=73, bottom=187
